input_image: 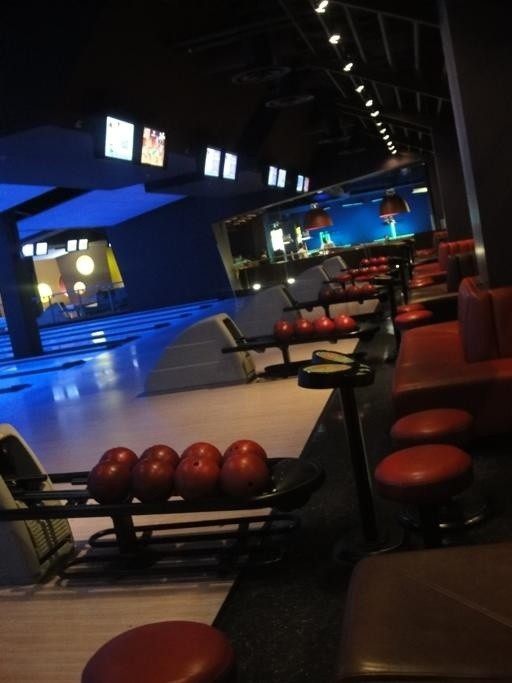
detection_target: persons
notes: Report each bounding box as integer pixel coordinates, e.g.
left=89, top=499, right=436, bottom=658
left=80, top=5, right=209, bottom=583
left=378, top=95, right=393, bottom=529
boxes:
left=297, top=243, right=309, bottom=260
left=260, top=249, right=268, bottom=261
left=318, top=229, right=335, bottom=255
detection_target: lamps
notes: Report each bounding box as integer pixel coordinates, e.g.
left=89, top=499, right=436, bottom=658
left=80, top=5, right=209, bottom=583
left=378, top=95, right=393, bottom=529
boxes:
left=379, top=186, right=410, bottom=219
left=303, top=200, right=332, bottom=230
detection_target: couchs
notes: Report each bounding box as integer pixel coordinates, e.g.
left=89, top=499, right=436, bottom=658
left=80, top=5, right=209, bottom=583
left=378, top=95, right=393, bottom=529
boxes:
left=390, top=275, right=512, bottom=408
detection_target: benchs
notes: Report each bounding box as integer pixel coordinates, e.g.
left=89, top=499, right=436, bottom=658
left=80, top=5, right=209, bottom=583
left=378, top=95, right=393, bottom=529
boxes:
left=336, top=540, right=511, bottom=683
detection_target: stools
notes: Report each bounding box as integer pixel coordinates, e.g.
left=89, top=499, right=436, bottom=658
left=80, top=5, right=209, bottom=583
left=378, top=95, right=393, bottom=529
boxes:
left=77, top=616, right=233, bottom=682
left=373, top=408, right=494, bottom=533
left=394, top=249, right=434, bottom=329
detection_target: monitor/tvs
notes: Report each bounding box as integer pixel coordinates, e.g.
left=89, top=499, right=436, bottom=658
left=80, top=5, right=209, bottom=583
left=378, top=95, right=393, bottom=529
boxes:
left=92, top=109, right=312, bottom=193
left=22, top=238, right=89, bottom=257
